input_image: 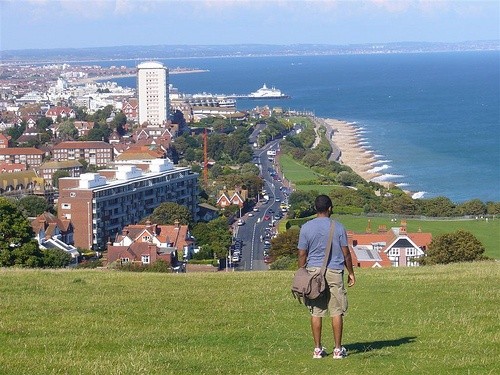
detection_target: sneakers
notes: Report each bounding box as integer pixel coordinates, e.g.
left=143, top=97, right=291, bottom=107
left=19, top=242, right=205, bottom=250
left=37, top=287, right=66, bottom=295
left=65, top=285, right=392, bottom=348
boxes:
left=312, top=346, right=328, bottom=358
left=333, top=346, right=348, bottom=360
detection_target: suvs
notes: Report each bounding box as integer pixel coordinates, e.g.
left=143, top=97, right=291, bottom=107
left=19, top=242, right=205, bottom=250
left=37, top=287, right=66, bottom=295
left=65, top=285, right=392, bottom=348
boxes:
left=275, top=197, right=280, bottom=202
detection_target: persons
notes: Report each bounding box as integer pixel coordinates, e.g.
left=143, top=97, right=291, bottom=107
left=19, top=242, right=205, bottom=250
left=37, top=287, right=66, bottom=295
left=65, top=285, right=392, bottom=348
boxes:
left=296, top=193, right=357, bottom=358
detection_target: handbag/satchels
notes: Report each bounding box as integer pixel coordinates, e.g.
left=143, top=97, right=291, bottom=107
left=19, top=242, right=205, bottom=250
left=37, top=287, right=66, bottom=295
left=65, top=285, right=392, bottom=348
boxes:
left=291, top=263, right=326, bottom=305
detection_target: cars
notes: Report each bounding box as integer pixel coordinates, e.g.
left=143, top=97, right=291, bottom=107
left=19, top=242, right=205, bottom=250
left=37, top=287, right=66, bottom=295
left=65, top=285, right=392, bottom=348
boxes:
left=268, top=168, right=282, bottom=182
left=263, top=227, right=271, bottom=263
left=274, top=201, right=288, bottom=220
left=268, top=222, right=275, bottom=227
left=248, top=213, right=253, bottom=217
left=238, top=219, right=244, bottom=226
left=253, top=207, right=258, bottom=212
left=261, top=190, right=270, bottom=204
left=231, top=238, right=243, bottom=262
